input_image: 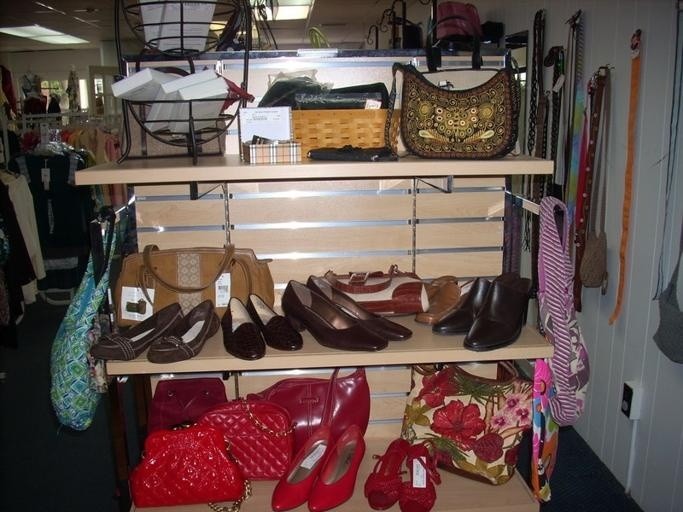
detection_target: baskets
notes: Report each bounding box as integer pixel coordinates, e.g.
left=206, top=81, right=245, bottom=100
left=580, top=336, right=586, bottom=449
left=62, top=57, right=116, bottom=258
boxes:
left=291, top=109, right=402, bottom=161
left=126, top=98, right=244, bottom=147
left=124, top=0, right=244, bottom=60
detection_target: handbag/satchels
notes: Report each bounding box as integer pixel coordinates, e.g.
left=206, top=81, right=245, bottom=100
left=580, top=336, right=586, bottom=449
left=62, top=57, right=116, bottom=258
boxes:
left=115, top=245, right=275, bottom=328
left=400, top=365, right=534, bottom=485
left=128, top=369, right=370, bottom=507
left=120, top=67, right=226, bottom=158
left=436, top=1, right=479, bottom=39
left=537, top=197, right=591, bottom=426
left=49, top=217, right=124, bottom=430
left=401, top=66, right=520, bottom=161
left=322, top=264, right=430, bottom=316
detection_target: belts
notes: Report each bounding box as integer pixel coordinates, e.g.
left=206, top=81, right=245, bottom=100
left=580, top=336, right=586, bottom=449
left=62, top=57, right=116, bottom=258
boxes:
left=523, top=10, right=607, bottom=311
left=609, top=29, right=641, bottom=323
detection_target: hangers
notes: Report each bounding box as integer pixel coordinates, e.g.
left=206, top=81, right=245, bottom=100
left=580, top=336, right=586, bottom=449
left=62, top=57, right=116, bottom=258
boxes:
left=0, top=115, right=121, bottom=186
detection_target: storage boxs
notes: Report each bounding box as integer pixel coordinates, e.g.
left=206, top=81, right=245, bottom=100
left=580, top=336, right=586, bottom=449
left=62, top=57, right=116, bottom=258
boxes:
left=242, top=140, right=302, bottom=167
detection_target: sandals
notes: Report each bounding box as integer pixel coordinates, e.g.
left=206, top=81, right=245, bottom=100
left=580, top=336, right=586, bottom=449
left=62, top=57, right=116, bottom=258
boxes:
left=399, top=440, right=441, bottom=512
left=364, top=438, right=409, bottom=509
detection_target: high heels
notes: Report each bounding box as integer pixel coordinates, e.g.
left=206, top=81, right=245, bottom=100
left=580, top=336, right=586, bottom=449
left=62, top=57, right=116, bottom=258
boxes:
left=306, top=275, right=412, bottom=342
left=281, top=280, right=389, bottom=351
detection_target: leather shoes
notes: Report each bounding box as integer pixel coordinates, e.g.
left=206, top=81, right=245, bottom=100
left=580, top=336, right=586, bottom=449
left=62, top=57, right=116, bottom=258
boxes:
left=246, top=293, right=304, bottom=351
left=415, top=279, right=477, bottom=325
left=427, top=276, right=459, bottom=300
left=272, top=426, right=336, bottom=511
left=464, top=272, right=524, bottom=351
left=431, top=276, right=491, bottom=335
left=220, top=296, right=266, bottom=360
left=309, top=425, right=365, bottom=510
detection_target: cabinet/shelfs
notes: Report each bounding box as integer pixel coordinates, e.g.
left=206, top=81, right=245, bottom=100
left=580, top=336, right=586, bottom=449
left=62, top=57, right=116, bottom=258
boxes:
left=73, top=54, right=556, bottom=510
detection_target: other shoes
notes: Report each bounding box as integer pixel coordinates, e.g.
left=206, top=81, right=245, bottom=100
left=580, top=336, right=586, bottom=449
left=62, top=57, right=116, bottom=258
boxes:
left=147, top=299, right=220, bottom=364
left=90, top=302, right=185, bottom=360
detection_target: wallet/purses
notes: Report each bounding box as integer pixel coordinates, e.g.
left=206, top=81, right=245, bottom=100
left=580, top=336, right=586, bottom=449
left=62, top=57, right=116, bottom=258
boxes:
left=307, top=147, right=397, bottom=161
